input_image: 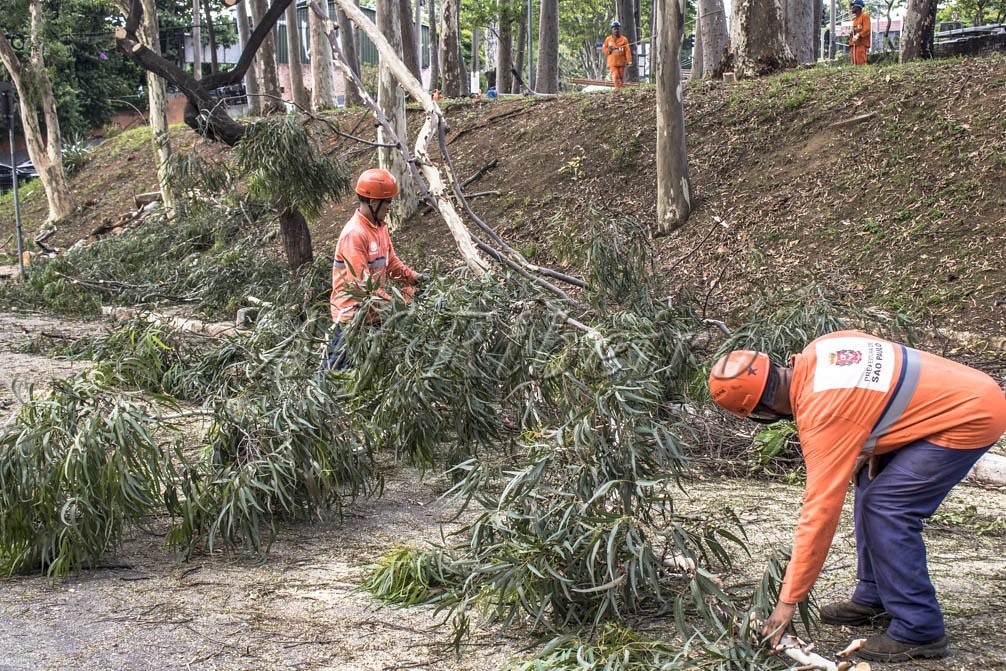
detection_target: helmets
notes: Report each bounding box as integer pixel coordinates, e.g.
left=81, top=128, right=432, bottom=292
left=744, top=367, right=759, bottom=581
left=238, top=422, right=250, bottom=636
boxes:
left=611, top=21, right=621, bottom=27
left=356, top=168, right=398, bottom=199
left=709, top=350, right=770, bottom=417
left=850, top=0, right=865, bottom=7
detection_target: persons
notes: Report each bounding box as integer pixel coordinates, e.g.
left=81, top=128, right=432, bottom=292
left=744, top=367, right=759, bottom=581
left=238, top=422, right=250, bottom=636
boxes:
left=602, top=21, right=632, bottom=89
left=708, top=329, right=1006, bottom=662
left=849, top=0, right=871, bottom=65
left=320, top=168, right=430, bottom=378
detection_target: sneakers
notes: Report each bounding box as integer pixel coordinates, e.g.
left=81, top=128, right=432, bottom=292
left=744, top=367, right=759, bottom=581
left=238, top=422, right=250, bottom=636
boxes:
left=820, top=597, right=886, bottom=626
left=858, top=631, right=947, bottom=662
